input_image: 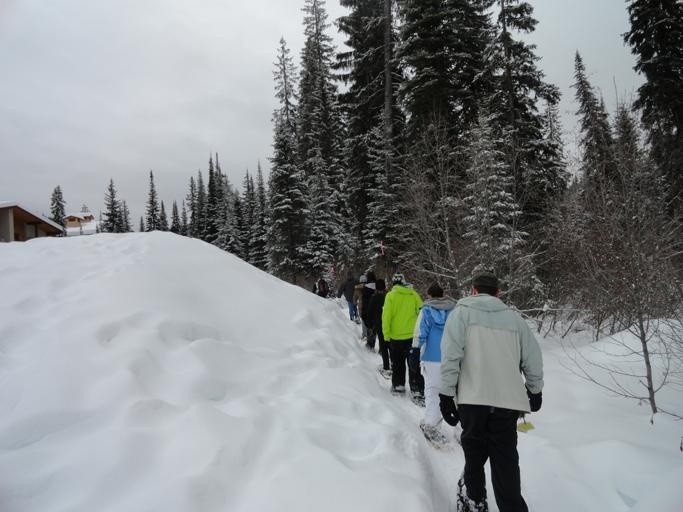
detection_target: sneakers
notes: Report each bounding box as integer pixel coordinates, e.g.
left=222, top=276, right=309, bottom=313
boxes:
left=377, top=364, right=393, bottom=380
left=360, top=333, right=367, bottom=342
left=419, top=423, right=447, bottom=445
left=456, top=476, right=489, bottom=512
left=365, top=342, right=375, bottom=353
left=408, top=388, right=426, bottom=407
left=390, top=385, right=406, bottom=397
left=349, top=316, right=361, bottom=325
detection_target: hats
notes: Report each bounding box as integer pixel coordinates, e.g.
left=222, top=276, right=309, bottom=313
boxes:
left=375, top=279, right=386, bottom=290
left=470, top=272, right=500, bottom=288
left=392, top=272, right=406, bottom=285
left=359, top=275, right=368, bottom=284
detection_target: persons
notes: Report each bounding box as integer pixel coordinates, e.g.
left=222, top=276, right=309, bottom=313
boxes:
left=359, top=270, right=377, bottom=351
left=437, top=270, right=545, bottom=511
left=312, top=278, right=330, bottom=299
left=380, top=272, right=425, bottom=407
left=406, top=284, right=464, bottom=446
left=337, top=270, right=361, bottom=324
left=365, top=278, right=395, bottom=376
left=351, top=275, right=369, bottom=339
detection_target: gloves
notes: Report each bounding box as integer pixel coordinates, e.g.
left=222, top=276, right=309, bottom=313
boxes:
left=368, top=327, right=375, bottom=337
left=385, top=341, right=393, bottom=350
left=524, top=384, right=543, bottom=412
left=407, top=348, right=421, bottom=375
left=438, top=393, right=460, bottom=427
